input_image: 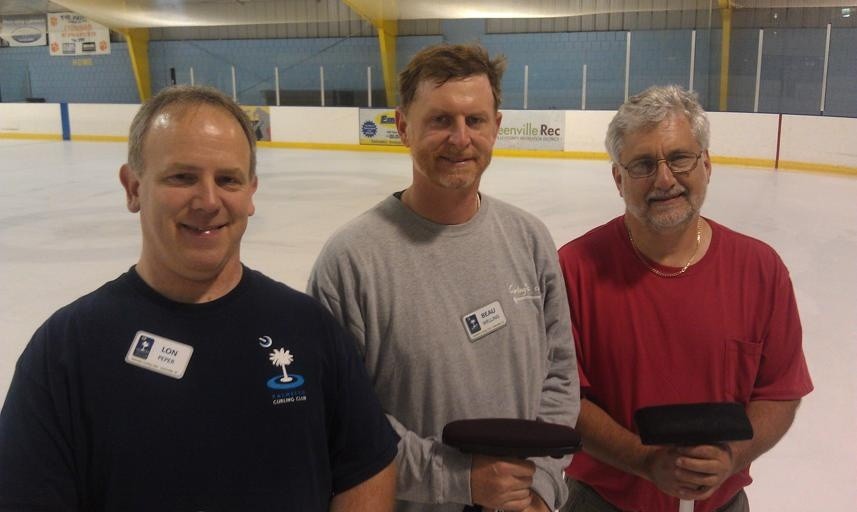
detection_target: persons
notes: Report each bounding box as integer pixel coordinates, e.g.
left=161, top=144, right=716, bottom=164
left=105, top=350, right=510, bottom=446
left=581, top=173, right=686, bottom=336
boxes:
left=552, top=83, right=814, bottom=512
left=0, top=84, right=402, bottom=512
left=306, top=43, right=581, bottom=512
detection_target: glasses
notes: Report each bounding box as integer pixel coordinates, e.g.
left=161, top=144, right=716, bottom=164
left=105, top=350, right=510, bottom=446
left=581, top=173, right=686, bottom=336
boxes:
left=619, top=151, right=702, bottom=179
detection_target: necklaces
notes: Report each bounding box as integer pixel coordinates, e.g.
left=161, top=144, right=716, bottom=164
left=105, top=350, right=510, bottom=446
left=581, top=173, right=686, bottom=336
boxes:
left=476, top=194, right=481, bottom=209
left=625, top=213, right=701, bottom=279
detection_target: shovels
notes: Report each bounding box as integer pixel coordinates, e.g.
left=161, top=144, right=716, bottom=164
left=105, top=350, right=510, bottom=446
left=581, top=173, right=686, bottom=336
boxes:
left=441, top=419, right=582, bottom=463
left=635, top=404, right=753, bottom=512
